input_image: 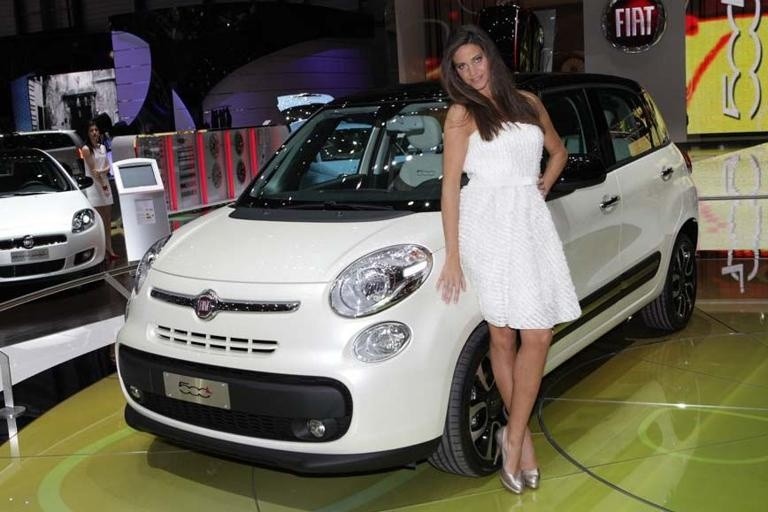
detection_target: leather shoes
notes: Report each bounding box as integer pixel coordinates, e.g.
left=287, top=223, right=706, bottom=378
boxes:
left=106, top=250, right=120, bottom=259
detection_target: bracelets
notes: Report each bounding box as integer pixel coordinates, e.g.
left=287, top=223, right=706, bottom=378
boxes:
left=102, top=185, right=108, bottom=191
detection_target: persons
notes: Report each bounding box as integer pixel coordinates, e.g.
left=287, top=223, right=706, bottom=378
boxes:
left=80, top=119, right=122, bottom=263
left=432, top=22, right=583, bottom=499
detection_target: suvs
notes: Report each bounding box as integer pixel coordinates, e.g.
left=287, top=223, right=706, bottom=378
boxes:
left=111, top=70, right=704, bottom=479
left=1, top=129, right=90, bottom=181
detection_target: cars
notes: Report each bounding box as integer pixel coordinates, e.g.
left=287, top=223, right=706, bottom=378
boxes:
left=1, top=145, right=109, bottom=302
left=276, top=93, right=415, bottom=192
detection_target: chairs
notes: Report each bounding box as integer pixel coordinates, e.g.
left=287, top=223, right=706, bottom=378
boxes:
left=381, top=116, right=639, bottom=191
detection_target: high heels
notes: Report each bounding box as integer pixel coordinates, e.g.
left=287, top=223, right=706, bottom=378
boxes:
left=520, top=468, right=540, bottom=489
left=493, top=426, right=526, bottom=494
